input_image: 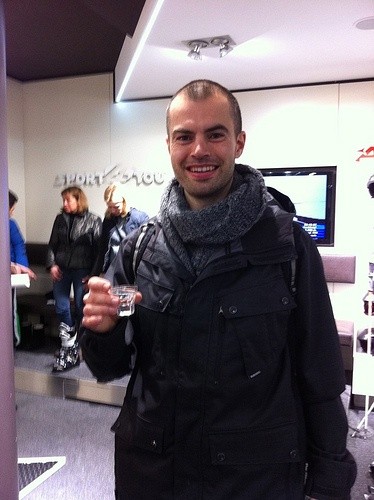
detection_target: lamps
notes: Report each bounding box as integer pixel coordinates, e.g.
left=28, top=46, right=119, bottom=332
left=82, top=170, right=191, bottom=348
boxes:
left=183, top=35, right=237, bottom=61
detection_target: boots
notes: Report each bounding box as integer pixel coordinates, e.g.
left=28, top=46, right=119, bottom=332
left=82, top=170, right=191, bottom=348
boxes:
left=53, top=322, right=79, bottom=370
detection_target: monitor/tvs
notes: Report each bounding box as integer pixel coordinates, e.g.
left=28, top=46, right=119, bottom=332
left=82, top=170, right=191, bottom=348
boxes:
left=258, top=166, right=336, bottom=247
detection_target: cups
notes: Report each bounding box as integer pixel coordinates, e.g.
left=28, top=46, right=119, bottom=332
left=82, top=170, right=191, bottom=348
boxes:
left=108, top=285, right=138, bottom=317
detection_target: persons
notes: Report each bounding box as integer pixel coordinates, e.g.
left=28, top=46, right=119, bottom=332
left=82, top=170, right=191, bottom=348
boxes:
left=9, top=182, right=158, bottom=372
left=76, top=80, right=357, bottom=500
